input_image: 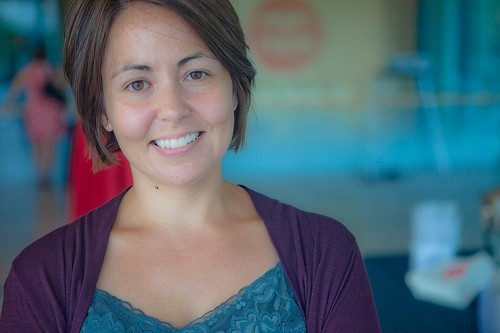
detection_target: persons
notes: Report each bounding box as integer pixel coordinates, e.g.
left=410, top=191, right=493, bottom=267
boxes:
left=7, top=41, right=61, bottom=186
left=0, top=0, right=378, bottom=333
